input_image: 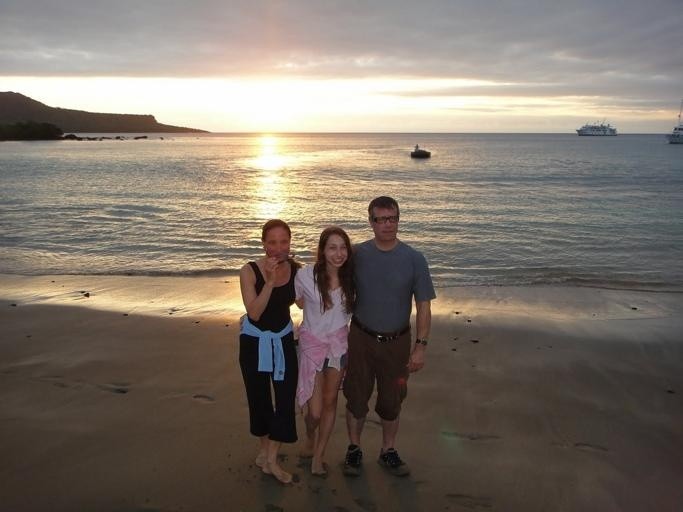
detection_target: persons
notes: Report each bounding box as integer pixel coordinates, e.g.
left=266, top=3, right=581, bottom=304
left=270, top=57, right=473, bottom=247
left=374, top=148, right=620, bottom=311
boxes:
left=343, top=197, right=439, bottom=480
left=239, top=220, right=306, bottom=485
left=294, top=225, right=361, bottom=475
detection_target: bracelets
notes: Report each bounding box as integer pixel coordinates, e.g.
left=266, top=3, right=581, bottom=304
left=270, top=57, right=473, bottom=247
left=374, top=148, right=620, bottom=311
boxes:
left=416, top=339, right=428, bottom=347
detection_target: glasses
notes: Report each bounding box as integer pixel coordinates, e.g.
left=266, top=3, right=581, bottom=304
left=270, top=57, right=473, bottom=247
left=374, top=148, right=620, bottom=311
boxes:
left=370, top=215, right=400, bottom=223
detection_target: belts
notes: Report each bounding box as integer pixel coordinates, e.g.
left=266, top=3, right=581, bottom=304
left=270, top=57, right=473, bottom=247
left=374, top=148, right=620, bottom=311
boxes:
left=351, top=316, right=411, bottom=343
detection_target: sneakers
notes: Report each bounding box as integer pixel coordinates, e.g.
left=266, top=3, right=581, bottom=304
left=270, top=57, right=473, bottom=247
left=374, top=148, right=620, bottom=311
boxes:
left=343, top=444, right=363, bottom=474
left=376, top=447, right=410, bottom=477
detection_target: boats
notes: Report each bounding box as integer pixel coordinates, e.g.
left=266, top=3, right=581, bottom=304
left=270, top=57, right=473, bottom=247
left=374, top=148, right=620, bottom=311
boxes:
left=575, top=120, right=616, bottom=136
left=410, top=144, right=430, bottom=159
left=664, top=99, right=683, bottom=145
